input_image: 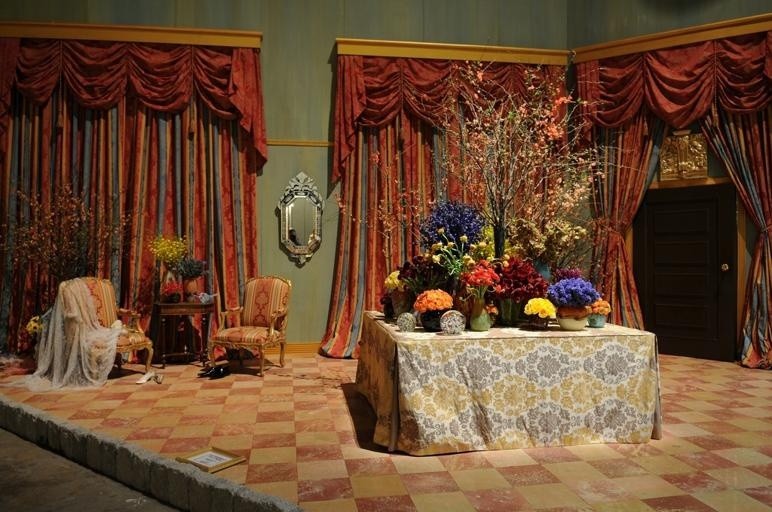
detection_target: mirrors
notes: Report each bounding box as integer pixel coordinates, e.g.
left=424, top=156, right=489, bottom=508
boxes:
left=280, top=169, right=324, bottom=265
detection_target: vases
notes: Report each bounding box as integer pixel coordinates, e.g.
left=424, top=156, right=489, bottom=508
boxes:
left=162, top=266, right=173, bottom=282
left=182, top=275, right=197, bottom=293
left=165, top=292, right=180, bottom=304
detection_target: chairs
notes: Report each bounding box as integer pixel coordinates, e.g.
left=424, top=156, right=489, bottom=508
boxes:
left=206, top=276, right=288, bottom=377
left=64, top=277, right=155, bottom=382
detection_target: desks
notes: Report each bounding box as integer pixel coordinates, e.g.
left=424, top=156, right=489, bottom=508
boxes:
left=136, top=291, right=215, bottom=366
left=353, top=308, right=663, bottom=458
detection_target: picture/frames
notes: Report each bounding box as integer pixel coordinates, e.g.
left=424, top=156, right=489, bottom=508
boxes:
left=178, top=443, right=248, bottom=476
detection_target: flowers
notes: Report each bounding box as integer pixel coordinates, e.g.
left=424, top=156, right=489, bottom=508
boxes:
left=179, top=255, right=209, bottom=278
left=2, top=180, right=140, bottom=299
left=161, top=280, right=183, bottom=296
left=26, top=315, right=46, bottom=338
left=143, top=228, right=190, bottom=269
left=384, top=54, right=611, bottom=333
